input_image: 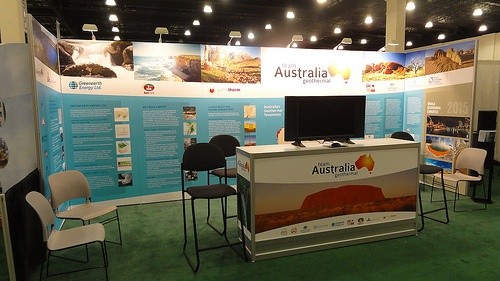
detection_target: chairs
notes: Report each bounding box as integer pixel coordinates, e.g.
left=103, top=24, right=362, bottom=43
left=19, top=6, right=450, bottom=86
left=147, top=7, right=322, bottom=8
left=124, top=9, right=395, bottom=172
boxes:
left=207, top=134, right=241, bottom=236
left=436, top=146, right=488, bottom=212
left=25, top=191, right=108, bottom=281
left=181, top=143, right=248, bottom=273
left=391, top=132, right=450, bottom=230
left=48, top=170, right=122, bottom=263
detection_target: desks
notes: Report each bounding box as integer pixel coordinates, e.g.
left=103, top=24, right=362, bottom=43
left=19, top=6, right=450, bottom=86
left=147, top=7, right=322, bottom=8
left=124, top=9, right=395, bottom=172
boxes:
left=236, top=137, right=421, bottom=262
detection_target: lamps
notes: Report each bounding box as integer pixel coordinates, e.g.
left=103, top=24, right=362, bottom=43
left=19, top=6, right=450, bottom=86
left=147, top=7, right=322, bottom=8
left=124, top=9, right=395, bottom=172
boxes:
left=227, top=31, right=241, bottom=46
left=286, top=35, right=303, bottom=48
left=155, top=27, right=169, bottom=43
left=82, top=24, right=98, bottom=40
left=333, top=38, right=352, bottom=50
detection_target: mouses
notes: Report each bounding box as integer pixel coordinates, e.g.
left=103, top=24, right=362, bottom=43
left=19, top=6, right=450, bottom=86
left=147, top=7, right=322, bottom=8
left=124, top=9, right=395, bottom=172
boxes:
left=332, top=143, right=341, bottom=147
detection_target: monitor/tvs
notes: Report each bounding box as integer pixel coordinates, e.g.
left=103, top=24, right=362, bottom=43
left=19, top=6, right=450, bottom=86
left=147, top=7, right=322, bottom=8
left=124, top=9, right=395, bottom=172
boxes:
left=284, top=95, right=366, bottom=147
